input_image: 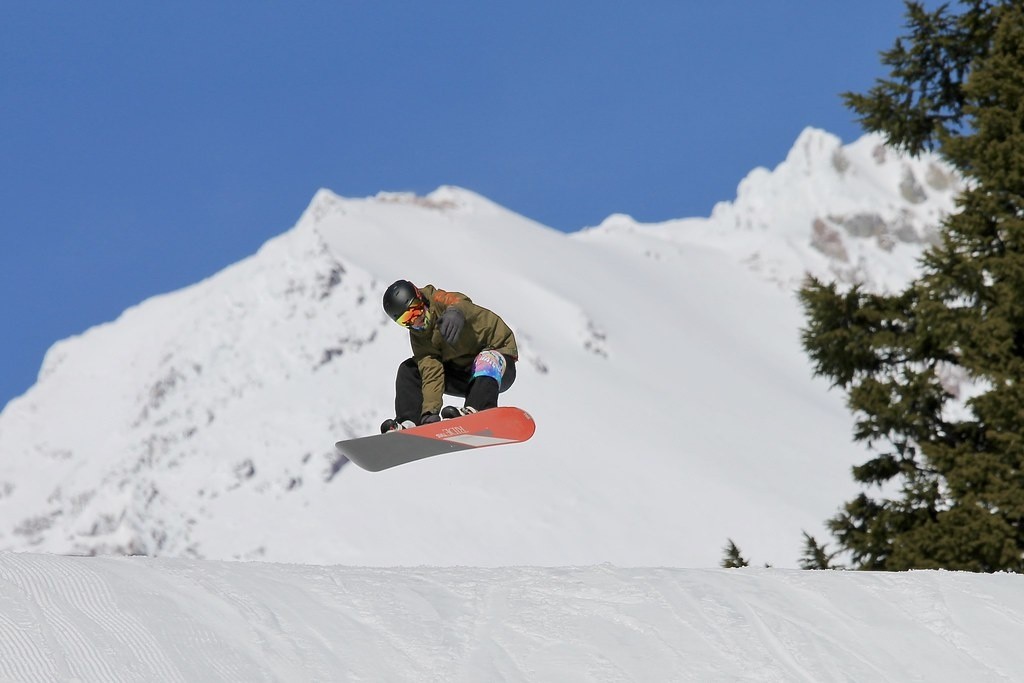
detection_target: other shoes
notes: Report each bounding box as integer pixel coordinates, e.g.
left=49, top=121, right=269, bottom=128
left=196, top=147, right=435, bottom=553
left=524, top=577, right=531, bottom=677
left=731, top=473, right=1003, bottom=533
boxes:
left=441, top=406, right=478, bottom=419
left=380, top=419, right=416, bottom=433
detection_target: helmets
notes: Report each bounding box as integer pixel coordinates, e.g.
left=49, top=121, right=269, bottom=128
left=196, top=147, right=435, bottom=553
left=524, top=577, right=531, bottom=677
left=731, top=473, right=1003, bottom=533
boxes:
left=383, top=280, right=429, bottom=329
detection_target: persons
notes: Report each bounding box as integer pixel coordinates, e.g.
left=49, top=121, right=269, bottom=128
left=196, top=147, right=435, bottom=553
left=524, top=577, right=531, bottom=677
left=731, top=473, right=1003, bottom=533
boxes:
left=381, top=280, right=518, bottom=434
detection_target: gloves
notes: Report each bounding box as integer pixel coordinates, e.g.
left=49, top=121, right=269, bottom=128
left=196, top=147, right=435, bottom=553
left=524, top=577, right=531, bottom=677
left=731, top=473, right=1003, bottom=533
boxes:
left=436, top=308, right=466, bottom=343
left=421, top=414, right=440, bottom=424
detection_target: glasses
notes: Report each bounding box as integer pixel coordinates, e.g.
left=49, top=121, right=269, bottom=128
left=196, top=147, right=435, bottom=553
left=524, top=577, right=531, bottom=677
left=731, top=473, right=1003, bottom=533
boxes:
left=396, top=297, right=424, bottom=327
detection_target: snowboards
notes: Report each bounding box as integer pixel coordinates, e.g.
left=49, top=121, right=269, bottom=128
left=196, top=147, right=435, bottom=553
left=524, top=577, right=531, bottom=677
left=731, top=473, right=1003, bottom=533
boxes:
left=334, top=406, right=536, bottom=473
